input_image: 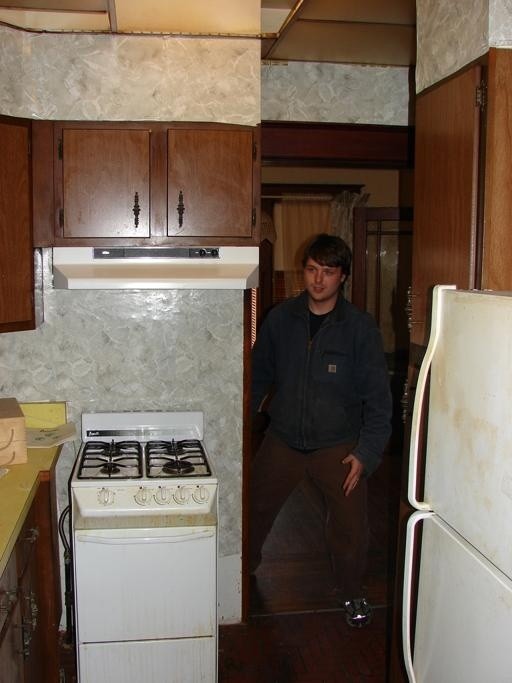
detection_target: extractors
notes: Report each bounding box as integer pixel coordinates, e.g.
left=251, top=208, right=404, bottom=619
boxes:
left=51, top=245, right=260, bottom=291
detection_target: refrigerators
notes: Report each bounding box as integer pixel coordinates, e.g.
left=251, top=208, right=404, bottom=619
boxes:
left=401, top=284, right=512, bottom=683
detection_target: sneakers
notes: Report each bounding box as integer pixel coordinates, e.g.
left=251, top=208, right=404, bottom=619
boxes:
left=340, top=595, right=373, bottom=629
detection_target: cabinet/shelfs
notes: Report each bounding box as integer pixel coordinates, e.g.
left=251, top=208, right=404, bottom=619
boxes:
left=51, top=116, right=263, bottom=249
left=0, top=471, right=63, bottom=683
left=0, top=109, right=46, bottom=334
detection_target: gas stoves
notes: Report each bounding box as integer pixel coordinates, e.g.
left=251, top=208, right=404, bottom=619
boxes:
left=69, top=437, right=218, bottom=516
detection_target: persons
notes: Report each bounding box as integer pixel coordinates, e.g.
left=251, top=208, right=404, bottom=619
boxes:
left=248, top=236, right=396, bottom=629
